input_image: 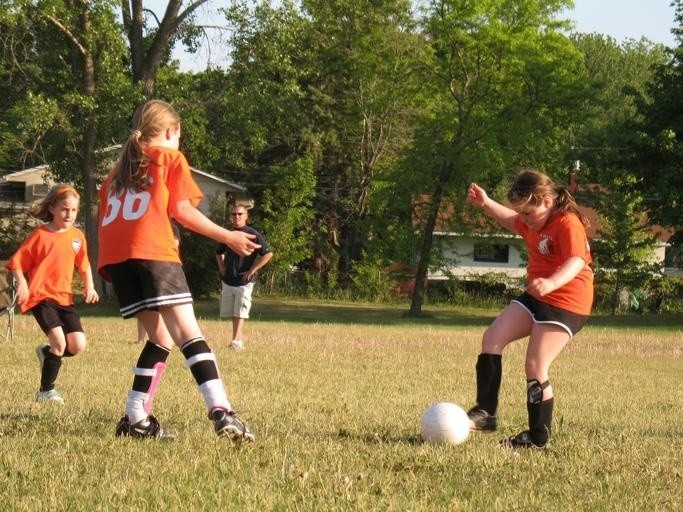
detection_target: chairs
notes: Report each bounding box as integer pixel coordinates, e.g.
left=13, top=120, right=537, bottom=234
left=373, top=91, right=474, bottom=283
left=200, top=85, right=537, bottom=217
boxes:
left=0, top=260, right=16, bottom=338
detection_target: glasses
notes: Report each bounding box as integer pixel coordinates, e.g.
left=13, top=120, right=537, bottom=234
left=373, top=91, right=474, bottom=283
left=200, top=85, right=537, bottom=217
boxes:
left=232, top=213, right=248, bottom=216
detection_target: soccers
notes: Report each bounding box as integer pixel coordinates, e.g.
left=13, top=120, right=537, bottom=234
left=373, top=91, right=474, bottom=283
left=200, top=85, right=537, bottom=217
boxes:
left=420, top=402, right=472, bottom=448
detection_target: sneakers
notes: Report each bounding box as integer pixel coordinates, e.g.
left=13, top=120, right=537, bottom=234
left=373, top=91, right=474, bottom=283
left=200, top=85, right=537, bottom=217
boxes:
left=212, top=410, right=254, bottom=442
left=37, top=389, right=63, bottom=403
left=230, top=340, right=242, bottom=350
left=499, top=430, right=547, bottom=448
left=466, top=405, right=497, bottom=430
left=37, top=343, right=51, bottom=370
left=116, top=416, right=161, bottom=438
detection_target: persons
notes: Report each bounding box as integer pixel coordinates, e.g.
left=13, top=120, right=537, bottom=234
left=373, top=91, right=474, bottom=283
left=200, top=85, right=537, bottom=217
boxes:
left=465, top=167, right=595, bottom=451
left=214, top=204, right=275, bottom=354
left=5, top=183, right=97, bottom=408
left=95, top=98, right=261, bottom=444
